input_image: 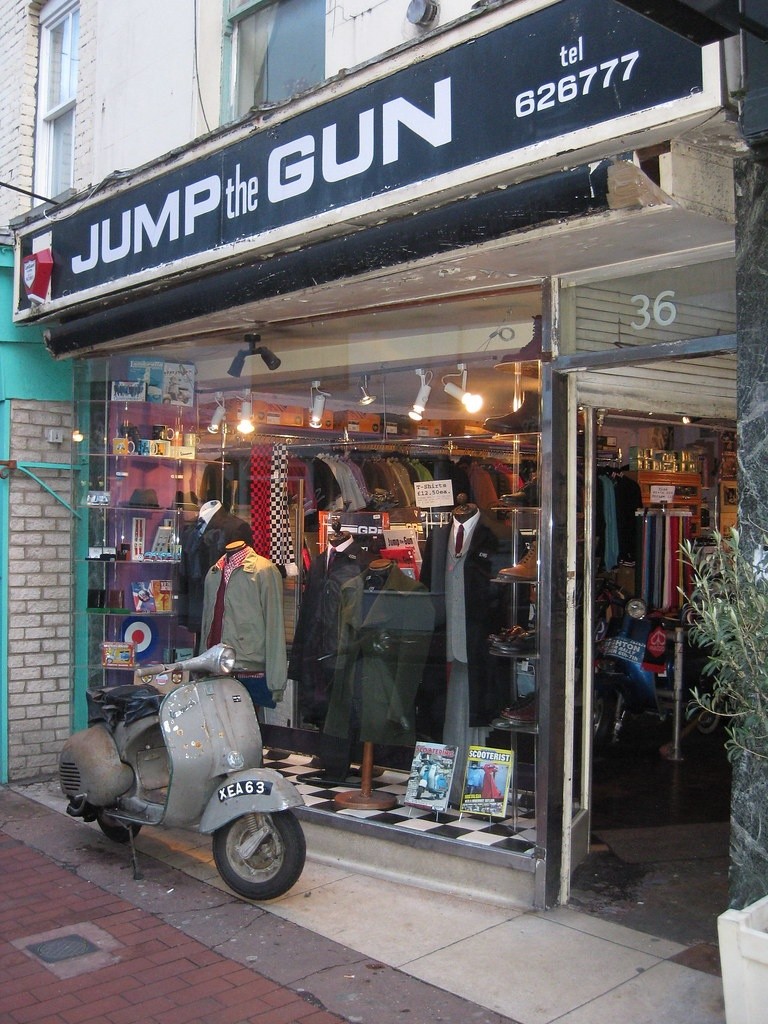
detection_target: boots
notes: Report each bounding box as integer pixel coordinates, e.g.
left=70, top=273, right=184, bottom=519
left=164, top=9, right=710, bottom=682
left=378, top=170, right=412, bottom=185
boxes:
left=484, top=390, right=541, bottom=433
left=497, top=540, right=538, bottom=581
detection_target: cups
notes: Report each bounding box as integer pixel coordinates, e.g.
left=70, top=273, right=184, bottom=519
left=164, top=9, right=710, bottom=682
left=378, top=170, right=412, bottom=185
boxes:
left=172, top=434, right=183, bottom=446
left=110, top=590, right=124, bottom=608
left=88, top=589, right=105, bottom=608
left=113, top=438, right=135, bottom=455
left=184, top=434, right=200, bottom=446
left=152, top=425, right=174, bottom=440
left=141, top=440, right=158, bottom=456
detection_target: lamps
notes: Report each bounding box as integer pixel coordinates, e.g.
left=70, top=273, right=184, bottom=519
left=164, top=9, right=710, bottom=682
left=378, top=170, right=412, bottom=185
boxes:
left=206, top=391, right=226, bottom=435
left=227, top=334, right=281, bottom=379
left=409, top=368, right=433, bottom=421
left=357, top=374, right=377, bottom=406
left=309, top=380, right=331, bottom=428
left=236, top=387, right=256, bottom=433
left=441, top=363, right=482, bottom=413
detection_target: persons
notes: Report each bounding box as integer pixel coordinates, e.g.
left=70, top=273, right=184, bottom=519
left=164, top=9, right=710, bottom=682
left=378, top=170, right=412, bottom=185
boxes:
left=289, top=521, right=371, bottom=691
left=199, top=542, right=288, bottom=708
left=323, top=559, right=435, bottom=745
left=421, top=495, right=508, bottom=745
left=178, top=500, right=255, bottom=630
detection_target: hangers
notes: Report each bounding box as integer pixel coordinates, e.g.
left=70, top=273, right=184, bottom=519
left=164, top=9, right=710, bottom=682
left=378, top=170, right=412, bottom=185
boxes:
left=243, top=434, right=536, bottom=466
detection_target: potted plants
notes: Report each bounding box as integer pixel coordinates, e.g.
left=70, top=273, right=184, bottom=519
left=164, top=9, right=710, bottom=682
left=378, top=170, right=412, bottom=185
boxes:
left=677, top=524, right=768, bottom=1023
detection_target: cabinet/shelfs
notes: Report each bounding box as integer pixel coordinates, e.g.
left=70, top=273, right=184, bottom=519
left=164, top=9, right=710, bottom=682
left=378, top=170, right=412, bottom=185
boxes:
left=91, top=454, right=230, bottom=691
left=622, top=470, right=702, bottom=539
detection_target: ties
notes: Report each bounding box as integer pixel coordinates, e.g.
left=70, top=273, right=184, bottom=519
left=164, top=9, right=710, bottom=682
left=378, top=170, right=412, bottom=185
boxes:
left=455, top=525, right=464, bottom=555
left=196, top=517, right=204, bottom=532
left=327, top=547, right=337, bottom=577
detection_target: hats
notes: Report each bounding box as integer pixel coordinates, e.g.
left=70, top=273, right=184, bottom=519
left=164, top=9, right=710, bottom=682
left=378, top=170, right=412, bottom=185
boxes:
left=122, top=488, right=164, bottom=509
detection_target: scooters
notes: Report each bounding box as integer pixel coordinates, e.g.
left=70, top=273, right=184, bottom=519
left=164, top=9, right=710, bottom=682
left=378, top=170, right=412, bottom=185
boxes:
left=596, top=575, right=729, bottom=748
left=58, top=639, right=308, bottom=903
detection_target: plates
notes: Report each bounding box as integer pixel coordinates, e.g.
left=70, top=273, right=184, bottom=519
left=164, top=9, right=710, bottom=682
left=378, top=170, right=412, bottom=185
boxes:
left=117, top=615, right=159, bottom=660
left=86, top=608, right=110, bottom=613
left=110, top=608, right=129, bottom=614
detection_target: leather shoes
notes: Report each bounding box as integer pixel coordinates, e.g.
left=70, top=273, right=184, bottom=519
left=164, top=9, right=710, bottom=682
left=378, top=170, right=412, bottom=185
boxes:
left=500, top=691, right=536, bottom=724
left=499, top=476, right=538, bottom=506
left=485, top=625, right=537, bottom=656
